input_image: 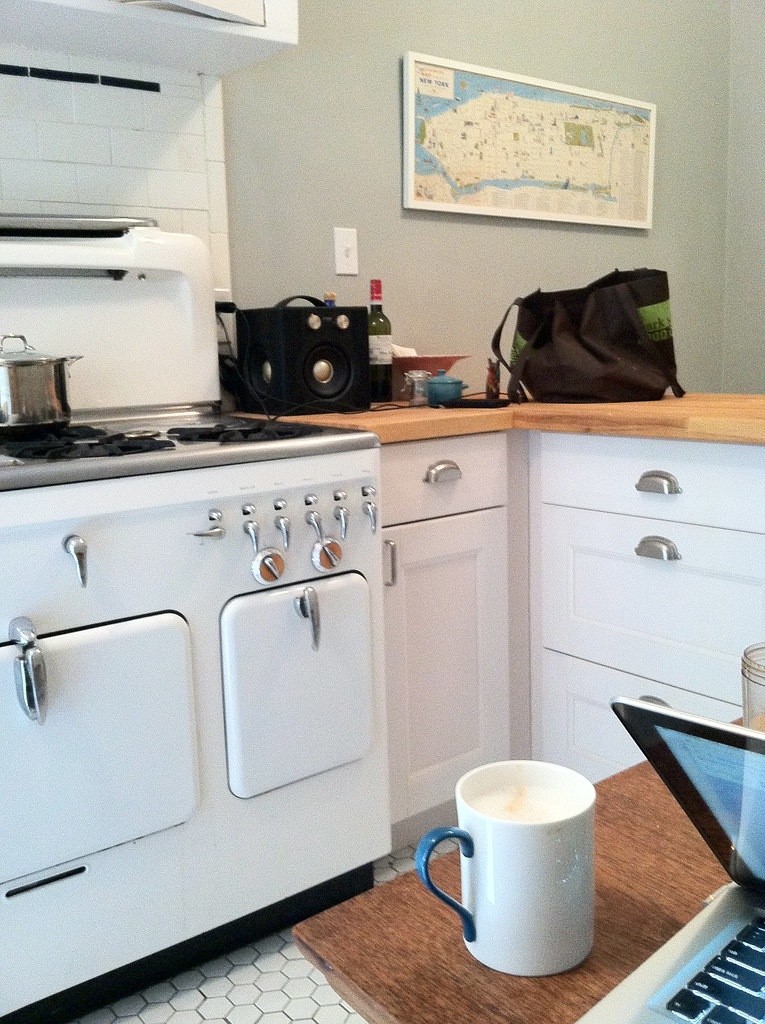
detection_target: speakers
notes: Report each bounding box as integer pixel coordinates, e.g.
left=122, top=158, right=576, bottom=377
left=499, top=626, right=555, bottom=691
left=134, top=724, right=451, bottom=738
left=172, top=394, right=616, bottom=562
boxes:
left=233, top=293, right=375, bottom=412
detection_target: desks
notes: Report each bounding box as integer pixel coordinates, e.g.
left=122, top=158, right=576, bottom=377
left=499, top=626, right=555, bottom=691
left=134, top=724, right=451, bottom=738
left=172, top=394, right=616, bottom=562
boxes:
left=289, top=712, right=762, bottom=1024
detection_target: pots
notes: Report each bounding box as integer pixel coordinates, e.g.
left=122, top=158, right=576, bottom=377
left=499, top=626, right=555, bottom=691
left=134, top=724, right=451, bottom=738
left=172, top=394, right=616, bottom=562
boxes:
left=0, top=334, right=84, bottom=437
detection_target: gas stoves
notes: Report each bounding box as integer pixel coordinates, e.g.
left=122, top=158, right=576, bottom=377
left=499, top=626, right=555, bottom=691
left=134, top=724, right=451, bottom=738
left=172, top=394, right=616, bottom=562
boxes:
left=0, top=411, right=365, bottom=472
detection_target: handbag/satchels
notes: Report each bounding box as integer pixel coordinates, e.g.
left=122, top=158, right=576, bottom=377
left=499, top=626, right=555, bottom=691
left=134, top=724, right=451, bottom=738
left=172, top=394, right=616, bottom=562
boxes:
left=490, top=266, right=690, bottom=404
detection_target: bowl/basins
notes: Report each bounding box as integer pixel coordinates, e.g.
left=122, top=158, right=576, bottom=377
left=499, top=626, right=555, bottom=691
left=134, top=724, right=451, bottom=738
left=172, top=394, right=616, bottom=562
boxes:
left=427, top=369, right=469, bottom=404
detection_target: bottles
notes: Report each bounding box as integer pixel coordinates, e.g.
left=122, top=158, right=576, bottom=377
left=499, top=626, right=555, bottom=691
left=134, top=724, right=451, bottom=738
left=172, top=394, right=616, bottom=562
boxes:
left=367, top=278, right=392, bottom=403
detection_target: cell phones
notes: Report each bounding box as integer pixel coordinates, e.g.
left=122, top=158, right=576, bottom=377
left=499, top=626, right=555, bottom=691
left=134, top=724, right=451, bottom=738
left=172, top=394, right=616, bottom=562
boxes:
left=441, top=398, right=510, bottom=408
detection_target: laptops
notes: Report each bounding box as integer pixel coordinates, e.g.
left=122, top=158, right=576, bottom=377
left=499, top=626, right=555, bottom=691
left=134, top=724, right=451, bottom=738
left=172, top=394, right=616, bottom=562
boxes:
left=573, top=698, right=765, bottom=1024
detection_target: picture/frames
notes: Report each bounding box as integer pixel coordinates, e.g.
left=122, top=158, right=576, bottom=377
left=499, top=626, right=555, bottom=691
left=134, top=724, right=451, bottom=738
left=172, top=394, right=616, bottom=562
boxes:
left=396, top=51, right=659, bottom=235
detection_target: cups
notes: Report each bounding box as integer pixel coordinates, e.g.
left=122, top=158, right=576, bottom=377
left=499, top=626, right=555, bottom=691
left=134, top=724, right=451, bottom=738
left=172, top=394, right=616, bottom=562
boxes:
left=404, top=370, right=431, bottom=405
left=741, top=641, right=765, bottom=733
left=413, top=759, right=596, bottom=978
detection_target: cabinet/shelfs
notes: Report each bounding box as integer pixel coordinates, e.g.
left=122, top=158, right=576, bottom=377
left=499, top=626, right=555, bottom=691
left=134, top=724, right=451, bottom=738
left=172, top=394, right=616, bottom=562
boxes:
left=380, top=430, right=515, bottom=857
left=515, top=425, right=765, bottom=784
left=2, top=0, right=298, bottom=81
